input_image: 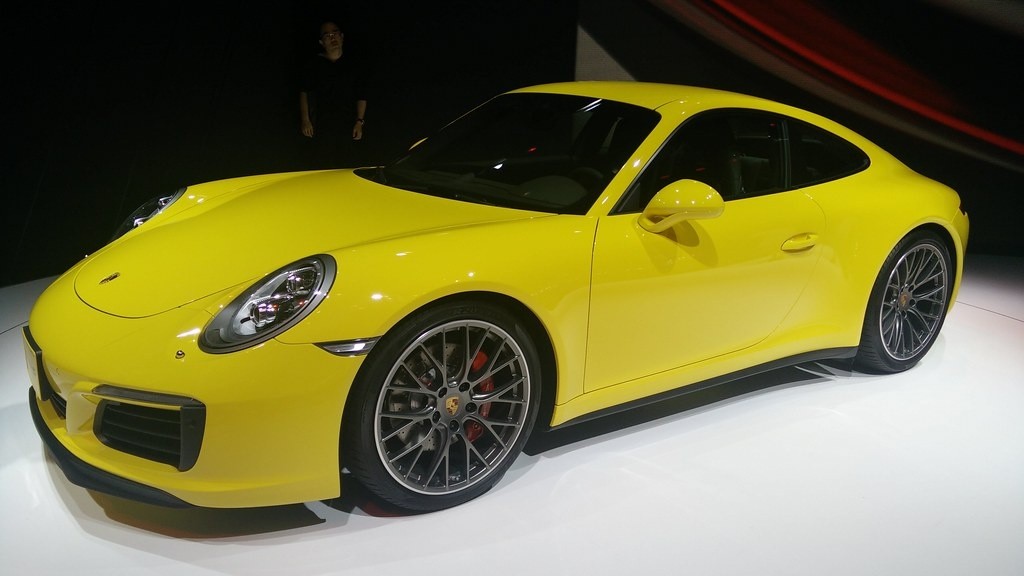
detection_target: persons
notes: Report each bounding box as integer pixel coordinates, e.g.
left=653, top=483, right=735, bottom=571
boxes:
left=297, top=20, right=369, bottom=167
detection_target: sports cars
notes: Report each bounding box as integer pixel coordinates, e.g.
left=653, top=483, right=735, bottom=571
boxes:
left=24, top=79, right=973, bottom=514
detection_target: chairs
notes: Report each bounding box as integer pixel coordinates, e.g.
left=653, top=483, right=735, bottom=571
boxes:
left=601, top=117, right=648, bottom=172
left=669, top=119, right=742, bottom=197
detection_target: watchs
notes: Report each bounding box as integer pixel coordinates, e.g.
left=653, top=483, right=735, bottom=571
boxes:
left=356, top=117, right=366, bottom=126
